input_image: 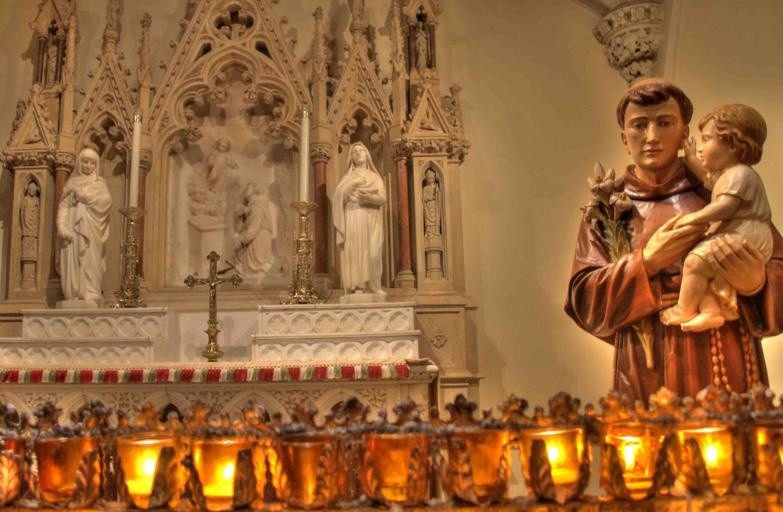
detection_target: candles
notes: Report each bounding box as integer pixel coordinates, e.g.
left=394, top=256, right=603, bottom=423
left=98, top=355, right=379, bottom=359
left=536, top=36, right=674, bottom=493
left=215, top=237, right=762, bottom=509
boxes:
left=300, top=105, right=310, bottom=200
left=128, top=109, right=141, bottom=207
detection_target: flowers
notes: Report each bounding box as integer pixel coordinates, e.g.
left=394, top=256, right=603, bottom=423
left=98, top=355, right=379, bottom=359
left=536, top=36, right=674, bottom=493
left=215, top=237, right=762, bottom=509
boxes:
left=580, top=159, right=656, bottom=371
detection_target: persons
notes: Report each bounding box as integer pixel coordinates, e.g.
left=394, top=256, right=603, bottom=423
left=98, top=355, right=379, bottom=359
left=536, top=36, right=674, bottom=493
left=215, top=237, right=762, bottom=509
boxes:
left=332, top=140, right=388, bottom=293
left=423, top=169, right=441, bottom=236
left=54, top=147, right=113, bottom=300
left=20, top=182, right=40, bottom=259
left=201, top=136, right=239, bottom=193
left=232, top=181, right=290, bottom=277
left=561, top=77, right=783, bottom=410
left=659, top=102, right=775, bottom=336
